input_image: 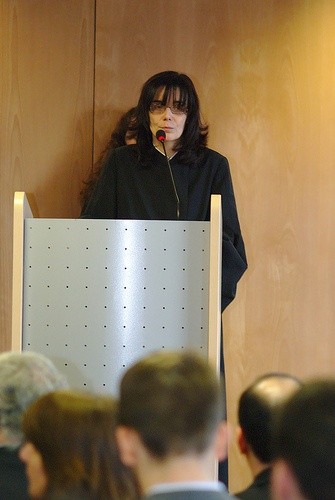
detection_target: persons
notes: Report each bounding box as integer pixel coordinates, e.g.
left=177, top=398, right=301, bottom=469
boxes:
left=78, top=69, right=248, bottom=493
left=113, top=350, right=242, bottom=500
left=0, top=351, right=68, bottom=500
left=231, top=372, right=335, bottom=500
left=19, top=391, right=144, bottom=500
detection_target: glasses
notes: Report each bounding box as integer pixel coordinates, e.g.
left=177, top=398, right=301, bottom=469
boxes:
left=148, top=100, right=189, bottom=115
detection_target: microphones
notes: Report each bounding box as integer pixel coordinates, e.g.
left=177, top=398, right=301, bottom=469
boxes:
left=157, top=130, right=180, bottom=220
